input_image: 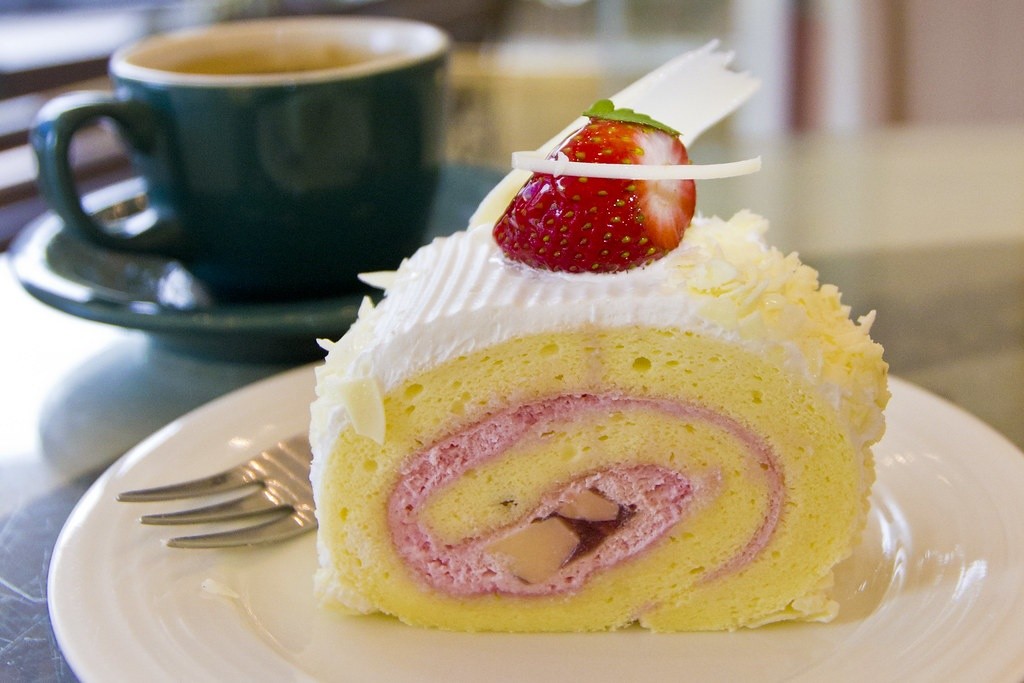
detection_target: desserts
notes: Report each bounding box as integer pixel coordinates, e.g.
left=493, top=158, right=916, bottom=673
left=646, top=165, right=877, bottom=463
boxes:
left=317, top=208, right=891, bottom=633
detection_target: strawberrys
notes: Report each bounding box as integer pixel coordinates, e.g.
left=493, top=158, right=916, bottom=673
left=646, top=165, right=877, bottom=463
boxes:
left=495, top=101, right=696, bottom=276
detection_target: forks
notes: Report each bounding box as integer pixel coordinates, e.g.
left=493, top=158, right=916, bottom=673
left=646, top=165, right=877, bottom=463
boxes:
left=117, top=425, right=318, bottom=550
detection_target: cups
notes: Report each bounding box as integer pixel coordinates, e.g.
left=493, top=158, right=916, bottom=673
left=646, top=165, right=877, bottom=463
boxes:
left=28, top=16, right=452, bottom=301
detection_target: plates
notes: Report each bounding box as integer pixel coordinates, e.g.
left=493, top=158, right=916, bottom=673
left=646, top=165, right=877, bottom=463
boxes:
left=9, top=165, right=513, bottom=356
left=46, top=357, right=1023, bottom=683
